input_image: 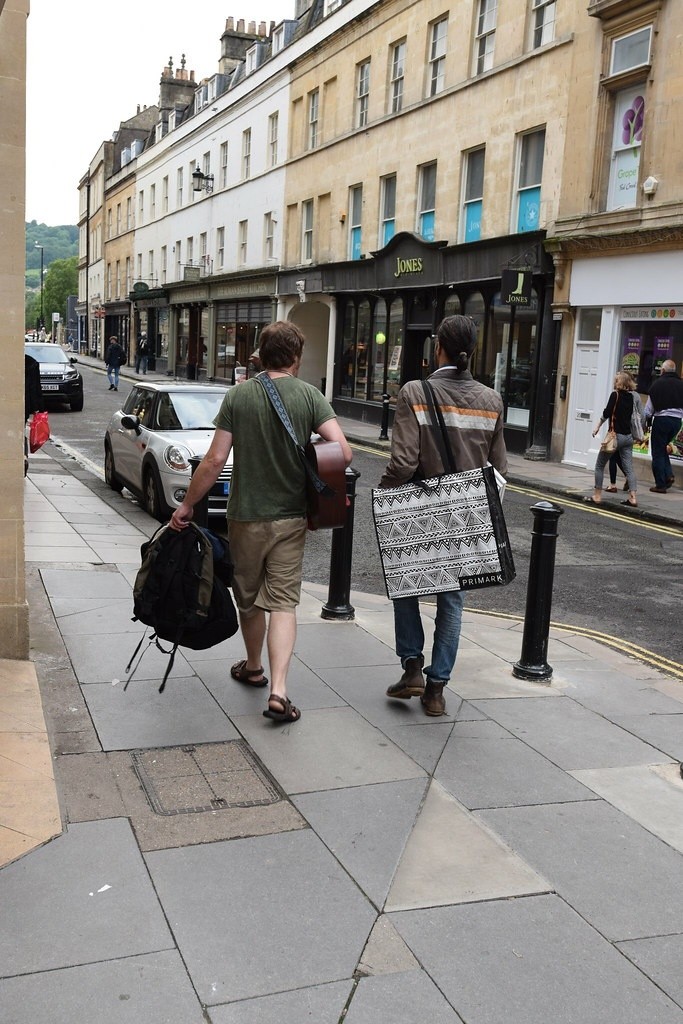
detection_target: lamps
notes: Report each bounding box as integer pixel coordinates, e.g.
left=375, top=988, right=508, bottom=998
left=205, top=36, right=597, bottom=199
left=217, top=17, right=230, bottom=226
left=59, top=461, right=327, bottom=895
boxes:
left=192, top=163, right=215, bottom=194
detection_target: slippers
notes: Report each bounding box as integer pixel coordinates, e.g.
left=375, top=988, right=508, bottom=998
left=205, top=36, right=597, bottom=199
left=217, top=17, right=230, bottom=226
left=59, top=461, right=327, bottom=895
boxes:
left=620, top=499, right=638, bottom=507
left=583, top=497, right=601, bottom=507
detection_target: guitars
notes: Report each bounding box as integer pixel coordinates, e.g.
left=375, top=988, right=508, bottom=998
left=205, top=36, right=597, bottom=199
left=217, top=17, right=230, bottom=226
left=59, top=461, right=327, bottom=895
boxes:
left=307, top=433, right=346, bottom=531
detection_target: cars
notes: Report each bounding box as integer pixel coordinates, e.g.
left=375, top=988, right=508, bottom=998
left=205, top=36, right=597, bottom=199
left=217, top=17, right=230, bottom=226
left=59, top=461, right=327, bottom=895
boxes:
left=103, top=381, right=235, bottom=526
left=25, top=329, right=52, bottom=343
left=218, top=343, right=235, bottom=362
left=24, top=341, right=84, bottom=412
left=248, top=348, right=265, bottom=372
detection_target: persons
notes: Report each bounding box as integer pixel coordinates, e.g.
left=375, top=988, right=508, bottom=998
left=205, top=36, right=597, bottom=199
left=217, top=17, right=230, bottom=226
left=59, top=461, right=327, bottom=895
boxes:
left=105, top=336, right=120, bottom=392
left=135, top=331, right=149, bottom=375
left=583, top=371, right=638, bottom=507
left=41, top=327, right=46, bottom=342
left=67, top=333, right=73, bottom=352
left=24, top=354, right=42, bottom=477
left=604, top=391, right=645, bottom=493
left=378, top=315, right=507, bottom=717
left=644, top=360, right=683, bottom=494
left=169, top=322, right=353, bottom=723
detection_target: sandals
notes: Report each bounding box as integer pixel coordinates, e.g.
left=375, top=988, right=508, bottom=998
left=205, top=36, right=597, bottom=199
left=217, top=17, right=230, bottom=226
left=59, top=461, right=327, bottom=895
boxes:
left=231, top=659, right=268, bottom=687
left=263, top=694, right=301, bottom=721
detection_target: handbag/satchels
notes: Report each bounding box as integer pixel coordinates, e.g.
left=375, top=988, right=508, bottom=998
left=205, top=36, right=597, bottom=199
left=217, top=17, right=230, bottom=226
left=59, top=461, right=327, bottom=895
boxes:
left=371, top=466, right=516, bottom=602
left=601, top=432, right=618, bottom=453
left=29, top=412, right=51, bottom=454
left=630, top=402, right=644, bottom=442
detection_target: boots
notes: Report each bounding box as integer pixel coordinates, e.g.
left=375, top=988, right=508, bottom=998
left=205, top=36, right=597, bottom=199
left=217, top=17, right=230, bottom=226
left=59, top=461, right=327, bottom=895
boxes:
left=420, top=678, right=446, bottom=716
left=386, top=657, right=425, bottom=699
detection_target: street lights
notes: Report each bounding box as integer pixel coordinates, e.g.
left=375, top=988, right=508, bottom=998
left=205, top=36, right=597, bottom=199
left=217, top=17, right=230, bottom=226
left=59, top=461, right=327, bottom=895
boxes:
left=34, top=244, right=44, bottom=326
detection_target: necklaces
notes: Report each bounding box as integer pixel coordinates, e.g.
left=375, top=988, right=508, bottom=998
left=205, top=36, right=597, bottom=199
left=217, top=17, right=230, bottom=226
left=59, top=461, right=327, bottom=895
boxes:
left=256, top=370, right=293, bottom=378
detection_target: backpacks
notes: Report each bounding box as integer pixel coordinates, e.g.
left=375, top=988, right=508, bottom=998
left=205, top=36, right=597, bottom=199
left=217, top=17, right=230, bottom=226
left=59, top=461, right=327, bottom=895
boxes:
left=123, top=520, right=240, bottom=693
left=108, top=346, right=127, bottom=367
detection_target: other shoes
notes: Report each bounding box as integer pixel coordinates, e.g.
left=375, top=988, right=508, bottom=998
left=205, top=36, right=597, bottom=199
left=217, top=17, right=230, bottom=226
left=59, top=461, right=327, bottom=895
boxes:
left=650, top=487, right=666, bottom=493
left=666, top=475, right=675, bottom=489
left=604, top=486, right=617, bottom=492
left=115, top=387, right=117, bottom=392
left=108, top=384, right=114, bottom=390
